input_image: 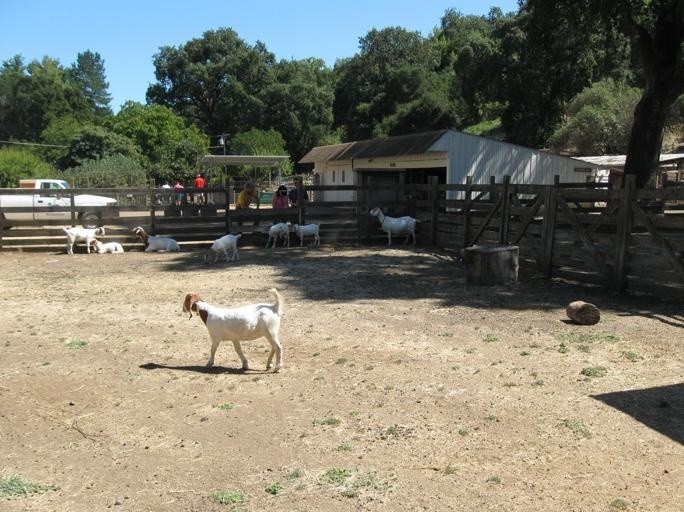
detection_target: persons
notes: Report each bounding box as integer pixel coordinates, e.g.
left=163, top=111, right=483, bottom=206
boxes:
left=235, top=183, right=260, bottom=234
left=194, top=174, right=204, bottom=204
left=161, top=180, right=170, bottom=188
left=272, top=185, right=288, bottom=224
left=288, top=177, right=308, bottom=225
left=173, top=181, right=183, bottom=205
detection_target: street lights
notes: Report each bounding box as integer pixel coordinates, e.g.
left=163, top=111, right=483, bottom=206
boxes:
left=220, top=136, right=225, bottom=154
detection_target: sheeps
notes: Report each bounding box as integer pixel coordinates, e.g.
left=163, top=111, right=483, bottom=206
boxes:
left=210, top=234, right=242, bottom=263
left=90, top=239, right=124, bottom=254
left=132, top=226, right=181, bottom=253
left=182, top=288, right=283, bottom=373
left=370, top=207, right=422, bottom=248
left=61, top=226, right=105, bottom=255
left=265, top=223, right=291, bottom=249
left=291, top=222, right=320, bottom=249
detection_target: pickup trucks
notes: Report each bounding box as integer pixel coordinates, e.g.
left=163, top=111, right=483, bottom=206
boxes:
left=0, top=179, right=119, bottom=230
